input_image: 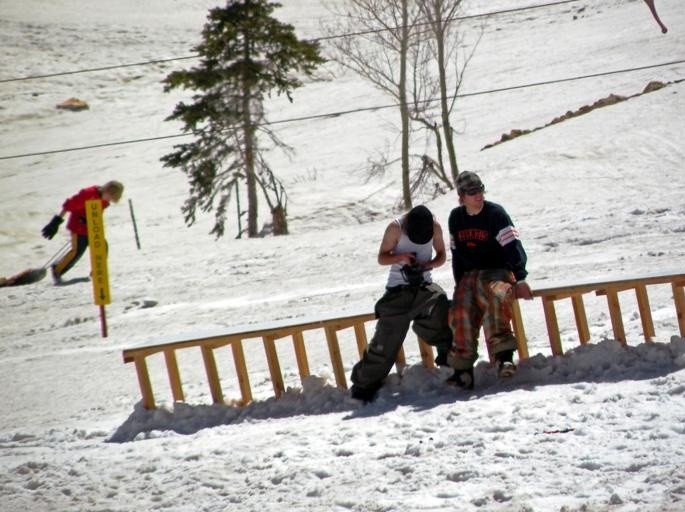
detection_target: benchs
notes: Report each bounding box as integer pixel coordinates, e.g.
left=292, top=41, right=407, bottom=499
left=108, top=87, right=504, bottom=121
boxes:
left=121, top=267, right=685, bottom=415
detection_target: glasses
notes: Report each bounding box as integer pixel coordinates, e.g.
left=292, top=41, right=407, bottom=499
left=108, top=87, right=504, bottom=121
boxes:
left=464, top=184, right=485, bottom=196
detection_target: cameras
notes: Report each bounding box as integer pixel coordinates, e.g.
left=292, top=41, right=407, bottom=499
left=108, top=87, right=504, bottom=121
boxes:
left=400, top=252, right=424, bottom=286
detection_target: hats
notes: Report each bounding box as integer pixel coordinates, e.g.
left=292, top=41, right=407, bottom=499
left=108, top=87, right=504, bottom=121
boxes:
left=406, top=206, right=435, bottom=244
left=104, top=181, right=123, bottom=203
left=455, top=171, right=483, bottom=195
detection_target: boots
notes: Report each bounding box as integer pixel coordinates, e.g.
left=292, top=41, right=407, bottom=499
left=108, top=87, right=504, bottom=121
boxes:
left=447, top=369, right=474, bottom=389
left=495, top=350, right=515, bottom=377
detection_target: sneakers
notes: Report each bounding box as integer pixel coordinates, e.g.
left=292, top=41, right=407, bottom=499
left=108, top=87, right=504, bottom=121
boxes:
left=434, top=350, right=449, bottom=366
left=351, top=382, right=381, bottom=402
left=51, top=263, right=63, bottom=283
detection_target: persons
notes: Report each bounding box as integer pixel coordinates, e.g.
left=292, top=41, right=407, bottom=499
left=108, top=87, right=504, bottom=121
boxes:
left=348, top=204, right=452, bottom=407
left=37, top=178, right=125, bottom=285
left=442, top=169, right=534, bottom=391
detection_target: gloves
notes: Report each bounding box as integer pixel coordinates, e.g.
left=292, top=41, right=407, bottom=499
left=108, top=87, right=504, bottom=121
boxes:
left=41, top=215, right=64, bottom=241
left=478, top=268, right=516, bottom=284
